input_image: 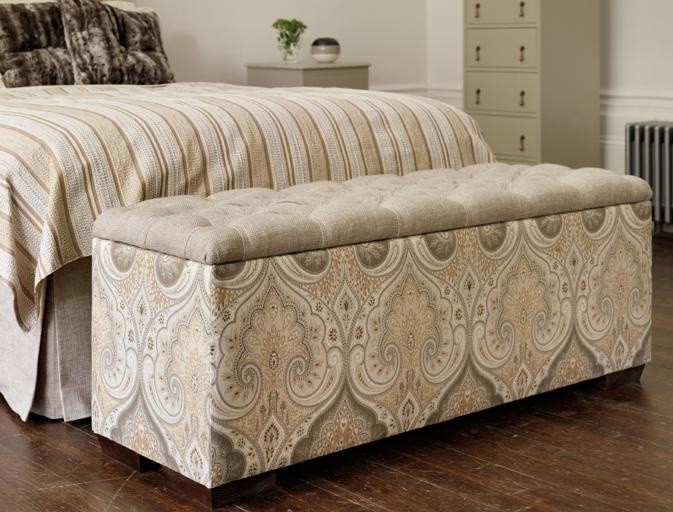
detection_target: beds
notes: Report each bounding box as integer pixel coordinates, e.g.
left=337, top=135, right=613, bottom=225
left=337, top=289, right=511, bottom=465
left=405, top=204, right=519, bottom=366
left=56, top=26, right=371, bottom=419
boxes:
left=0, top=3, right=500, bottom=427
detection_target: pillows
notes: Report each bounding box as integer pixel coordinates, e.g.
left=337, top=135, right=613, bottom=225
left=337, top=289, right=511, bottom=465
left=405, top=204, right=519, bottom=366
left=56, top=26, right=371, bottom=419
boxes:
left=0, top=2, right=74, bottom=88
left=60, top=0, right=175, bottom=83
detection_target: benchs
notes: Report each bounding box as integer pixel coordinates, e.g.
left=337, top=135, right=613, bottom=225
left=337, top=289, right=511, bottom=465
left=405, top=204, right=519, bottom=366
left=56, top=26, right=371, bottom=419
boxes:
left=90, top=155, right=654, bottom=510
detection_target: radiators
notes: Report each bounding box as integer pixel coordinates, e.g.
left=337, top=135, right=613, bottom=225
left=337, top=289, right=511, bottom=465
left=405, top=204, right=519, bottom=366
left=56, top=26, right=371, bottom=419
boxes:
left=623, top=120, right=672, bottom=236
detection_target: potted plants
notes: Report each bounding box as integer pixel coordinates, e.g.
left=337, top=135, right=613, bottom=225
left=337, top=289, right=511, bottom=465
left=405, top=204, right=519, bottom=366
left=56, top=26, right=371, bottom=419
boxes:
left=271, top=16, right=307, bottom=62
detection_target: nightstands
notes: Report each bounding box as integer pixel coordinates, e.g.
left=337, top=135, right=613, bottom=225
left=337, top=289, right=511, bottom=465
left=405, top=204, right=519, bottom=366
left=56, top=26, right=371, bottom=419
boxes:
left=245, top=58, right=374, bottom=90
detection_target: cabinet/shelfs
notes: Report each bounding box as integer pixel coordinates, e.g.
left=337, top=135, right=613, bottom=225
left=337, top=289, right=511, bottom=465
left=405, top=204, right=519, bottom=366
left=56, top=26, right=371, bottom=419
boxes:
left=462, top=1, right=600, bottom=170
